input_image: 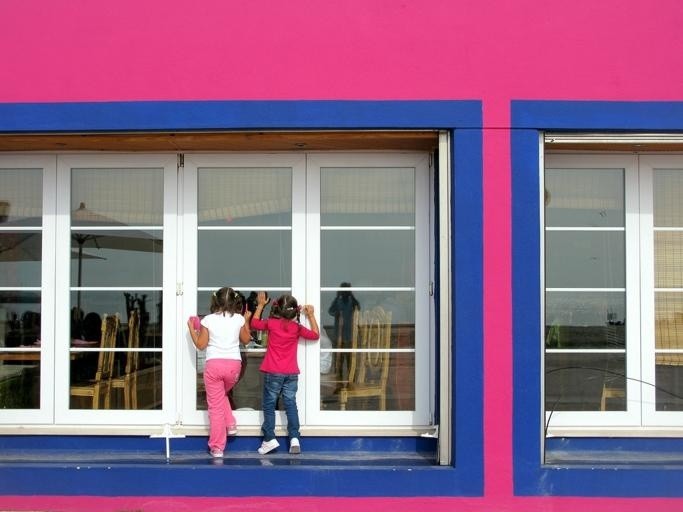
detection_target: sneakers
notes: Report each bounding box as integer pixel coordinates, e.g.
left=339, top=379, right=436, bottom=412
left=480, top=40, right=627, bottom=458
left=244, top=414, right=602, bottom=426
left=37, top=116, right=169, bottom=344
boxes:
left=210, top=447, right=223, bottom=457
left=227, top=425, right=238, bottom=435
left=289, top=437, right=301, bottom=454
left=257, top=438, right=280, bottom=455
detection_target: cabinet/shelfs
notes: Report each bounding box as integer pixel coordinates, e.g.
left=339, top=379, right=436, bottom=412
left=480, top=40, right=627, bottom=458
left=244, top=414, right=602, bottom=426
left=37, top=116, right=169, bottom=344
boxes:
left=117, top=369, right=162, bottom=409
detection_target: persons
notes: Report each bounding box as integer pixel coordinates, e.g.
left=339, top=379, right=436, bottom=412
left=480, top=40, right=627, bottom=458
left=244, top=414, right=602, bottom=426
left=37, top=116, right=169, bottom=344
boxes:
left=249, top=289, right=321, bottom=455
left=242, top=291, right=261, bottom=347
left=1, top=304, right=126, bottom=407
left=185, top=287, right=253, bottom=459
left=327, top=281, right=360, bottom=396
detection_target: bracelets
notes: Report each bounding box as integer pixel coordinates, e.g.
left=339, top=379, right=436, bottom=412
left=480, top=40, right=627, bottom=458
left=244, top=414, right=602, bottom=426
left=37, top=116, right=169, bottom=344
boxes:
left=255, top=306, right=263, bottom=310
left=308, top=313, right=314, bottom=319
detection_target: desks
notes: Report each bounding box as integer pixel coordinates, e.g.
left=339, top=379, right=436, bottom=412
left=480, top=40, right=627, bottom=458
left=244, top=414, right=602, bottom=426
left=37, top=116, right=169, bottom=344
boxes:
left=0, top=344, right=97, bottom=366
left=231, top=352, right=265, bottom=409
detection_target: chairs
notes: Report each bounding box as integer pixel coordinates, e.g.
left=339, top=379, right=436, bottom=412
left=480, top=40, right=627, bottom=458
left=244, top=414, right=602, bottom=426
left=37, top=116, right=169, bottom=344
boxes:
left=320, top=305, right=392, bottom=410
left=71, top=311, right=140, bottom=409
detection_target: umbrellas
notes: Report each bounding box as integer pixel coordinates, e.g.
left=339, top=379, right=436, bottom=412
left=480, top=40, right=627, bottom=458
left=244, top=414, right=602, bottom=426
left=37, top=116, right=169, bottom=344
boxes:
left=0, top=244, right=105, bottom=265
left=0, top=202, right=163, bottom=335
